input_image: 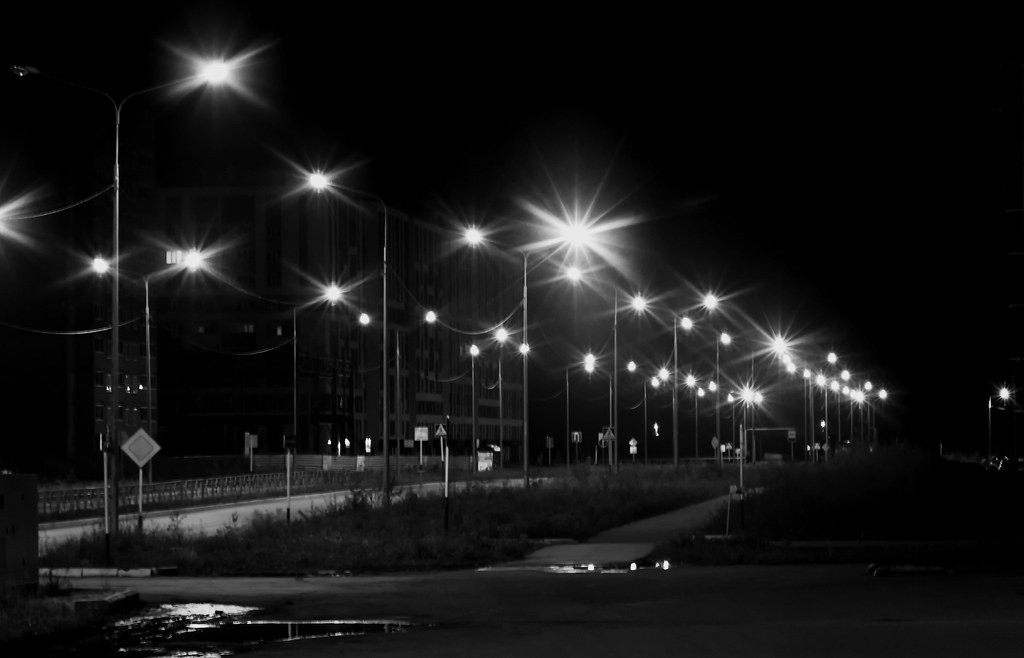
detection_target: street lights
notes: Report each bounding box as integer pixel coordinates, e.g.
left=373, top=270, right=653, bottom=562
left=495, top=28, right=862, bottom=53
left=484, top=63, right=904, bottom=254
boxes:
left=0, top=42, right=1016, bottom=580
